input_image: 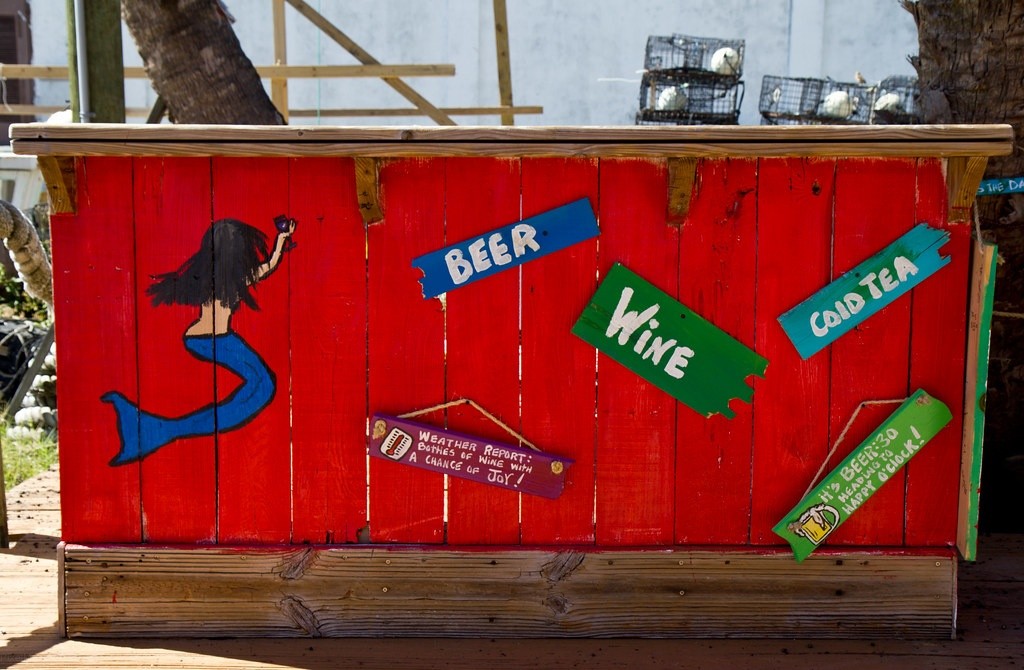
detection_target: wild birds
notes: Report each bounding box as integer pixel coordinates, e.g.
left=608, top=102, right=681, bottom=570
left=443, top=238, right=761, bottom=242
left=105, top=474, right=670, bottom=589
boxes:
left=856, top=72, right=867, bottom=84
left=656, top=47, right=739, bottom=111
left=819, top=91, right=906, bottom=119
